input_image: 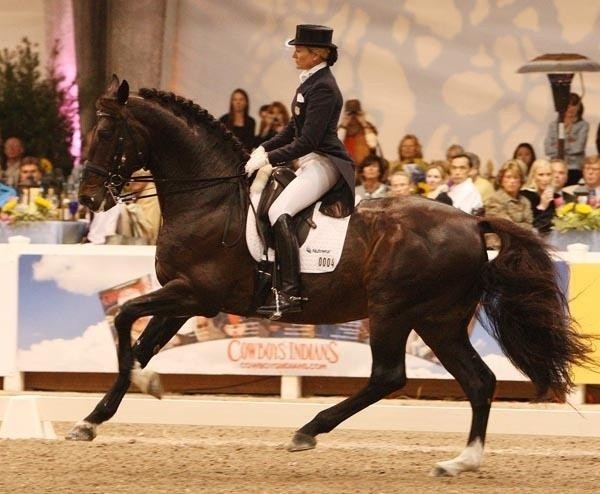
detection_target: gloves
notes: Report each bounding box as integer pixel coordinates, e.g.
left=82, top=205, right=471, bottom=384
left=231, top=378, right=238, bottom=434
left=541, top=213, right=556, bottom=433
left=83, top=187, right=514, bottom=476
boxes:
left=245, top=145, right=269, bottom=178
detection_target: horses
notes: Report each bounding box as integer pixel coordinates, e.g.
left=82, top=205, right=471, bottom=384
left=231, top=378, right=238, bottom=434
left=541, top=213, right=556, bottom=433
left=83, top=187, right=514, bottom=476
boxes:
left=64, top=73, right=600, bottom=478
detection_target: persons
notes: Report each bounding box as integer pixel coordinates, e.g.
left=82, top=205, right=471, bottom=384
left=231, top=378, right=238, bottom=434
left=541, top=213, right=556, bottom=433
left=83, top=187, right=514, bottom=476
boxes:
left=571, top=155, right=599, bottom=207
left=218, top=89, right=290, bottom=153
left=485, top=143, right=568, bottom=235
left=0, top=138, right=61, bottom=209
left=544, top=94, right=589, bottom=187
left=416, top=145, right=495, bottom=214
left=244, top=24, right=357, bottom=316
left=354, top=155, right=416, bottom=206
left=337, top=100, right=377, bottom=165
left=390, top=135, right=427, bottom=172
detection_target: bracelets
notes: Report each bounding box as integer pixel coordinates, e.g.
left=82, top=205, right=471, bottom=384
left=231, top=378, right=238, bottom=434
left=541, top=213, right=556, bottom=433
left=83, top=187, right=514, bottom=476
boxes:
left=339, top=125, right=347, bottom=129
left=363, top=125, right=370, bottom=129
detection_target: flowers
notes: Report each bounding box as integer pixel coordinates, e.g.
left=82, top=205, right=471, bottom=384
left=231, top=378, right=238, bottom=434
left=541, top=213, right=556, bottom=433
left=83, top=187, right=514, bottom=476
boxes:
left=548, top=200, right=600, bottom=232
left=2, top=194, right=55, bottom=221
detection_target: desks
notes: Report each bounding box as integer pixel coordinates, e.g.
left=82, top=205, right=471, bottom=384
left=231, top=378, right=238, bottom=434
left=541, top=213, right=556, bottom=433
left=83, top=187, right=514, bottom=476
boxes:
left=1, top=214, right=92, bottom=243
left=545, top=229, right=599, bottom=250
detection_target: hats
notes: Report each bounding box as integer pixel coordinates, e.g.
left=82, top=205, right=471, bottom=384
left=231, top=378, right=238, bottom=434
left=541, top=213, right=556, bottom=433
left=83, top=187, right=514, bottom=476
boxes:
left=289, top=25, right=337, bottom=47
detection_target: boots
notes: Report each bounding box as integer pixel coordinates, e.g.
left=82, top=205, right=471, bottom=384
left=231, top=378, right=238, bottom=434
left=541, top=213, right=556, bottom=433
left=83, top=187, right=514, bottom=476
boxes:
left=257, top=213, right=304, bottom=316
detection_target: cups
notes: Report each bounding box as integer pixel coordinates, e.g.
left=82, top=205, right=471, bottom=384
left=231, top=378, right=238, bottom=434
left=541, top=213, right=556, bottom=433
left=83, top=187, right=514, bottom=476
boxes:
left=44, top=193, right=78, bottom=222
